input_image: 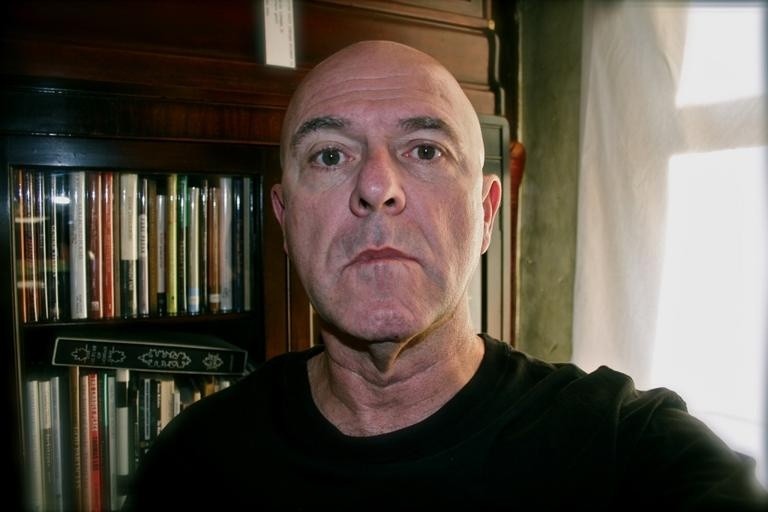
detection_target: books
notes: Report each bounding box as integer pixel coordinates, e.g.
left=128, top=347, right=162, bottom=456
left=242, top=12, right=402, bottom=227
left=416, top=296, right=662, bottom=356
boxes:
left=24, top=335, right=256, bottom=512
left=12, top=170, right=263, bottom=324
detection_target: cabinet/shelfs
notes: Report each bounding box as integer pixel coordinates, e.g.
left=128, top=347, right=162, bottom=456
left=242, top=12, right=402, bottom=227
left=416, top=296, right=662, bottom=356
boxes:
left=0, top=2, right=509, bottom=509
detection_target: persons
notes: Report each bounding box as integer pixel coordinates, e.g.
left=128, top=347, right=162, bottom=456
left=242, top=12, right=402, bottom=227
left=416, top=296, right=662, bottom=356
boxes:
left=121, top=39, right=768, bottom=511
